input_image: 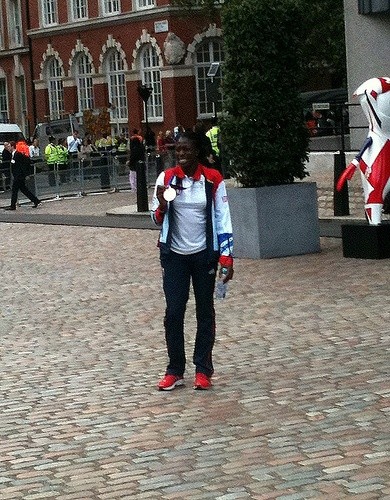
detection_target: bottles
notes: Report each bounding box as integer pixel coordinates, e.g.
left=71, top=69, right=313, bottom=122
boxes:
left=216, top=268, right=228, bottom=300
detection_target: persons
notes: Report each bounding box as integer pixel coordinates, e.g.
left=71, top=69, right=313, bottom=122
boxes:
left=5, top=141, right=41, bottom=210
left=45, top=130, right=130, bottom=186
left=126, top=129, right=146, bottom=203
left=304, top=102, right=350, bottom=137
left=29, top=139, right=41, bottom=174
left=143, top=124, right=192, bottom=179
left=150, top=133, right=234, bottom=391
left=16, top=135, right=31, bottom=175
left=190, top=116, right=231, bottom=179
left=0, top=142, right=12, bottom=193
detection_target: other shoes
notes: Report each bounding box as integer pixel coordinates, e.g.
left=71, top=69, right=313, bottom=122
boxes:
left=5, top=206, right=16, bottom=210
left=157, top=374, right=184, bottom=391
left=193, top=373, right=212, bottom=390
left=32, top=198, right=41, bottom=208
